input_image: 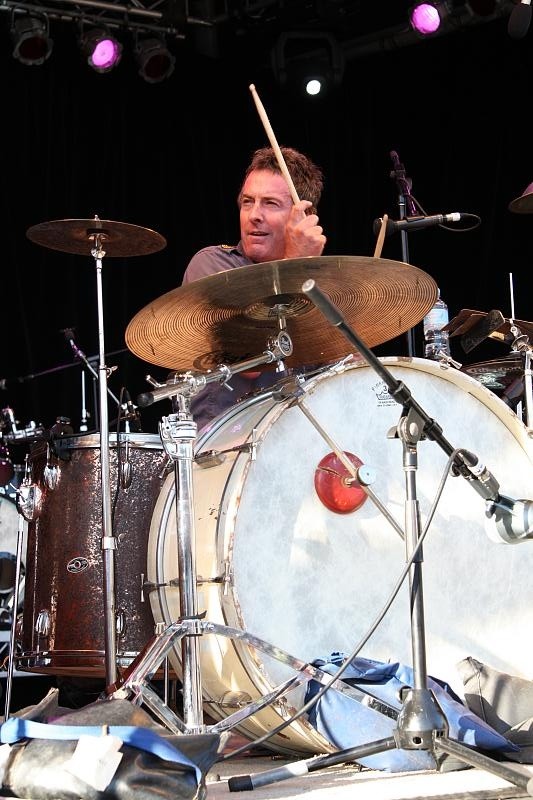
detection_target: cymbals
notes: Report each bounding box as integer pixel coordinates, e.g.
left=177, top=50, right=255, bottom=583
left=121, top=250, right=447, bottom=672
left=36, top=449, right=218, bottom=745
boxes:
left=124, top=256, right=438, bottom=375
left=25, top=218, right=167, bottom=258
left=460, top=355, right=524, bottom=388
left=507, top=192, right=533, bottom=214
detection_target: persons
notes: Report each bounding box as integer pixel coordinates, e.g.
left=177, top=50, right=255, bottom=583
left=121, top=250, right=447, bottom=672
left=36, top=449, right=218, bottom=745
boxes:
left=181, top=145, right=363, bottom=760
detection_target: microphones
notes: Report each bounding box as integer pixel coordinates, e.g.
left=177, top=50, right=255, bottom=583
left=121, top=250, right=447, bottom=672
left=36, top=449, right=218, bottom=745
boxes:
left=124, top=390, right=142, bottom=432
left=372, top=213, right=469, bottom=237
left=485, top=499, right=533, bottom=544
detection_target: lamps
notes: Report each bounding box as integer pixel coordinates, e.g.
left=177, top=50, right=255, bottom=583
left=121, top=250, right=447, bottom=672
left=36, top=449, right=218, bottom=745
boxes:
left=128, top=30, right=177, bottom=85
left=75, top=13, right=123, bottom=74
left=405, top=0, right=475, bottom=38
left=10, top=4, right=53, bottom=67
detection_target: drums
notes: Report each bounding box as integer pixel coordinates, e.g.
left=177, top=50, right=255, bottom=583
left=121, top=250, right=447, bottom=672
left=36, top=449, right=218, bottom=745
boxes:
left=16, top=432, right=178, bottom=681
left=3, top=464, right=27, bottom=498
left=4, top=428, right=35, bottom=465
left=146, top=355, right=533, bottom=758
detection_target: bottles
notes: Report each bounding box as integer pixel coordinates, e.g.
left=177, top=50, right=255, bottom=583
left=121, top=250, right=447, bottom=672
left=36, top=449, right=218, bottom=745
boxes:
left=424, top=287, right=451, bottom=362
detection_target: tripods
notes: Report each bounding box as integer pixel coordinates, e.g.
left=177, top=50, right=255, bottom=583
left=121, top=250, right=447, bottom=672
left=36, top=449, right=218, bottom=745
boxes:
left=111, top=280, right=533, bottom=797
